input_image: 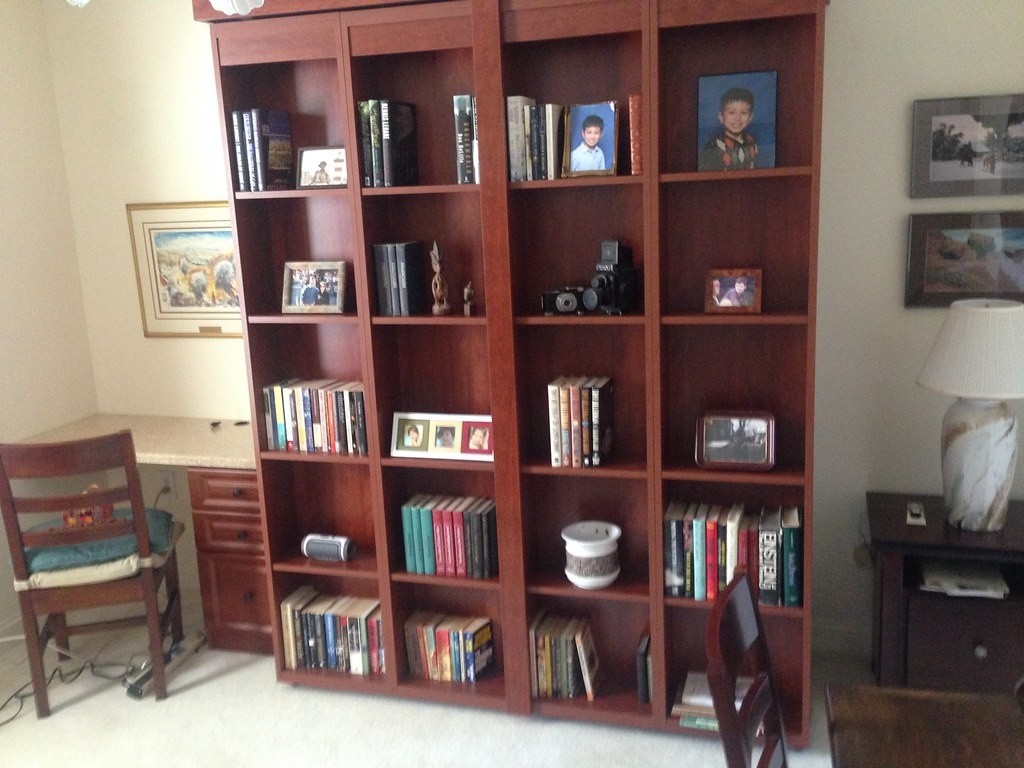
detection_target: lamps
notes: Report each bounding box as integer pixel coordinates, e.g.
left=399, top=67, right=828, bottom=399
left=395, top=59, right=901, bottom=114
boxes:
left=916, top=298, right=1023, bottom=532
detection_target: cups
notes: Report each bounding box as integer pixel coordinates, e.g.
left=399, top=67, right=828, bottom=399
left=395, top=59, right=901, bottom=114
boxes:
left=561, top=519, right=623, bottom=590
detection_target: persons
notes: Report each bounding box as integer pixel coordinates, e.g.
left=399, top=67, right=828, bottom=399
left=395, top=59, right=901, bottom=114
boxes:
left=290, top=270, right=330, bottom=306
left=468, top=427, right=488, bottom=450
left=712, top=277, right=749, bottom=306
left=405, top=425, right=422, bottom=447
left=437, top=427, right=454, bottom=448
left=698, top=87, right=757, bottom=171
left=570, top=115, right=606, bottom=172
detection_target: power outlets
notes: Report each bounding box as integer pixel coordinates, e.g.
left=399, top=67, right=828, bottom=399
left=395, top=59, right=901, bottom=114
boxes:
left=159, top=472, right=176, bottom=500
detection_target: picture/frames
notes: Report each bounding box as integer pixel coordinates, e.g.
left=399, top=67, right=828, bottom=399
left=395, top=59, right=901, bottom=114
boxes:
left=699, top=71, right=777, bottom=174
left=280, top=262, right=347, bottom=314
left=910, top=92, right=1024, bottom=198
left=390, top=412, right=492, bottom=461
left=126, top=201, right=243, bottom=339
left=706, top=268, right=762, bottom=313
left=694, top=408, right=777, bottom=471
left=560, top=101, right=620, bottom=179
left=297, top=145, right=349, bottom=190
left=904, top=211, right=1024, bottom=310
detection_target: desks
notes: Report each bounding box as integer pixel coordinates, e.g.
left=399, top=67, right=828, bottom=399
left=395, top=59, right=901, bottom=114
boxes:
left=18, top=412, right=274, bottom=655
left=825, top=681, right=1024, bottom=768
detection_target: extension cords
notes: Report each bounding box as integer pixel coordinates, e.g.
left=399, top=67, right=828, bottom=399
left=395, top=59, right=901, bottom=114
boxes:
left=126, top=632, right=207, bottom=701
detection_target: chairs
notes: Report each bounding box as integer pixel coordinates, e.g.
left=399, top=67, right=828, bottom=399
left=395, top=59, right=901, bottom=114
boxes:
left=705, top=574, right=789, bottom=768
left=1, top=428, right=184, bottom=719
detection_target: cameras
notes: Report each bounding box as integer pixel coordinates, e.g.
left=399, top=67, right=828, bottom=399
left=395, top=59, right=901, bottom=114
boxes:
left=541, top=286, right=587, bottom=315
left=582, top=240, right=638, bottom=316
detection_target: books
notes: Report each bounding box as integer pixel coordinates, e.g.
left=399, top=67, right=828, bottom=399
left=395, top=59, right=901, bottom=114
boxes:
left=917, top=559, right=1009, bottom=598
left=230, top=108, right=294, bottom=192
left=528, top=607, right=605, bottom=700
left=634, top=633, right=652, bottom=704
left=452, top=95, right=480, bottom=185
left=279, top=585, right=384, bottom=676
left=356, top=99, right=419, bottom=188
left=670, top=670, right=765, bottom=735
left=662, top=500, right=802, bottom=608
left=403, top=609, right=493, bottom=683
left=506, top=95, right=562, bottom=182
left=373, top=240, right=429, bottom=317
left=546, top=375, right=614, bottom=468
left=264, top=378, right=368, bottom=455
left=400, top=492, right=497, bottom=579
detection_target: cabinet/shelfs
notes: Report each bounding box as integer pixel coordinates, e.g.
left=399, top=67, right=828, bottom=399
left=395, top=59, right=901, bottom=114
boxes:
left=192, top=0, right=830, bottom=750
left=866, top=493, right=1024, bottom=695
